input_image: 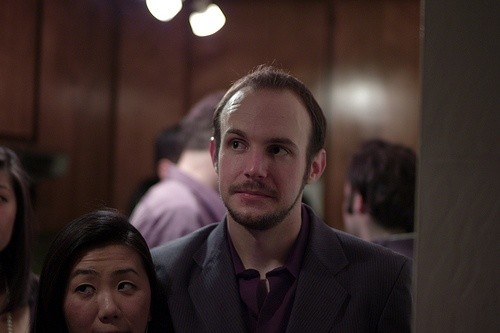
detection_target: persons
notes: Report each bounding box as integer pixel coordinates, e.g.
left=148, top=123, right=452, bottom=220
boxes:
left=148, top=60, right=414, bottom=333
left=342, top=134, right=418, bottom=251
left=129, top=124, right=185, bottom=215
left=30, top=207, right=176, bottom=333
left=126, top=89, right=228, bottom=249
left=0, top=147, right=43, bottom=333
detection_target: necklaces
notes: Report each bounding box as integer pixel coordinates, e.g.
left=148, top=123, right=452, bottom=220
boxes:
left=5, top=278, right=14, bottom=333
